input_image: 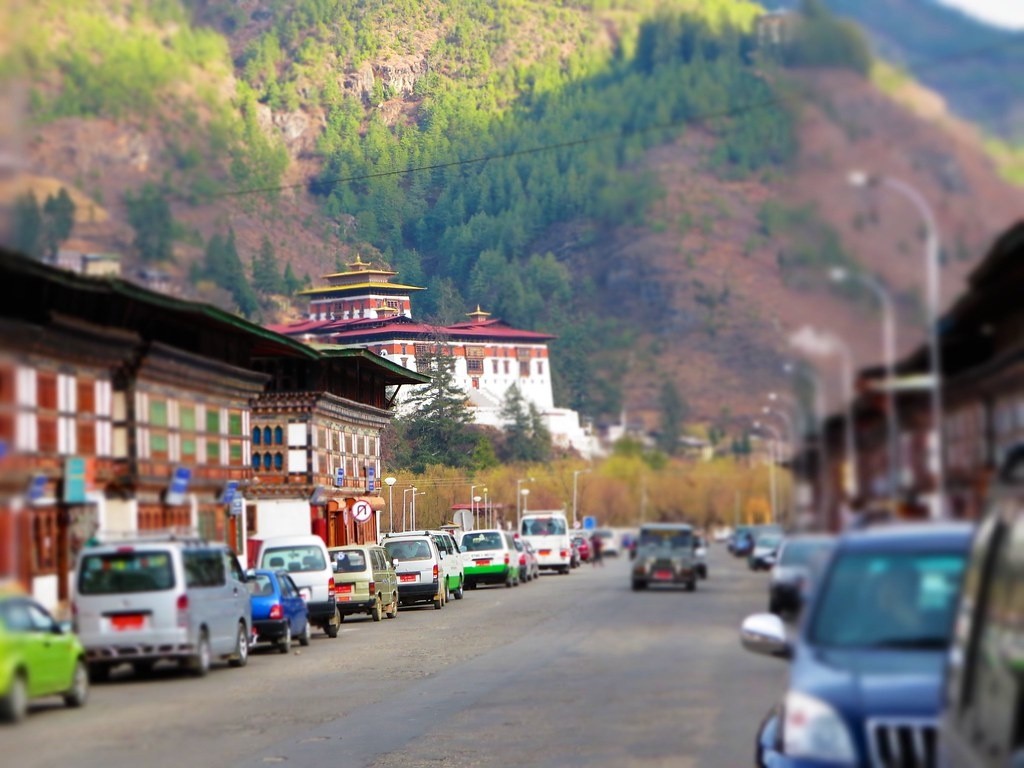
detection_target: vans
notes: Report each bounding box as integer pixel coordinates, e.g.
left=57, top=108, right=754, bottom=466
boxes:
left=327, top=546, right=399, bottom=622
left=457, top=529, right=520, bottom=590
left=251, top=533, right=341, bottom=640
left=520, top=514, right=570, bottom=574
left=382, top=530, right=445, bottom=609
left=389, top=530, right=465, bottom=604
left=68, top=529, right=256, bottom=683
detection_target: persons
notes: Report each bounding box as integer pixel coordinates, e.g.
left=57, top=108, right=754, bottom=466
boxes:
left=589, top=532, right=603, bottom=567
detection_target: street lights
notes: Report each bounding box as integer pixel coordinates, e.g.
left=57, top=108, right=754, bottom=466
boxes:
left=743, top=332, right=854, bottom=520
left=828, top=267, right=899, bottom=489
left=847, top=168, right=943, bottom=443
left=240, top=477, right=260, bottom=570
left=413, top=492, right=426, bottom=531
left=517, top=479, right=535, bottom=527
left=402, top=488, right=418, bottom=531
left=573, top=468, right=593, bottom=523
left=521, top=489, right=530, bottom=510
left=471, top=484, right=486, bottom=516
left=385, top=477, right=396, bottom=532
left=483, top=487, right=488, bottom=529
left=473, top=496, right=482, bottom=530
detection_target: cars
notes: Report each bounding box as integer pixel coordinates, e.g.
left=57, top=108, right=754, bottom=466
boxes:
left=730, top=527, right=774, bottom=554
left=0, top=594, right=89, bottom=723
left=748, top=534, right=783, bottom=572
left=693, top=530, right=710, bottom=580
left=588, top=530, right=621, bottom=557
left=630, top=536, right=636, bottom=561
left=762, top=538, right=831, bottom=614
left=737, top=520, right=971, bottom=768
left=570, top=537, right=581, bottom=569
left=936, top=442, right=1024, bottom=768
left=244, top=568, right=311, bottom=655
left=572, top=536, right=593, bottom=562
left=515, top=537, right=539, bottom=581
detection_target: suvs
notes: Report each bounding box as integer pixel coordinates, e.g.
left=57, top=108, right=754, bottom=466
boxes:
left=631, top=524, right=696, bottom=593
